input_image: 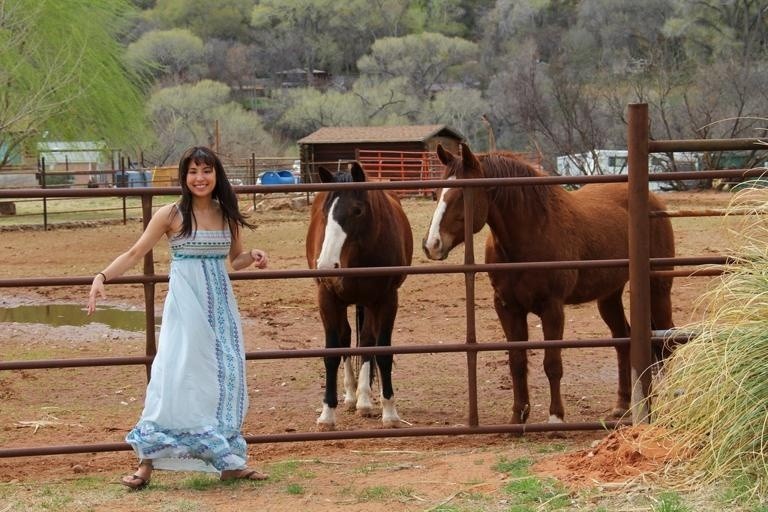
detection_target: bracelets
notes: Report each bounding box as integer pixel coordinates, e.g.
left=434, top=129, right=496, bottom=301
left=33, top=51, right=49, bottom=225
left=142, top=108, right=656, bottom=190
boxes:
left=249, top=248, right=254, bottom=259
left=91, top=270, right=105, bottom=285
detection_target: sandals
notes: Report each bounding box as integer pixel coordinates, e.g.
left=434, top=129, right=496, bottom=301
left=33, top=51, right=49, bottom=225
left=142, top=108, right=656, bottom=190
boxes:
left=120, top=462, right=154, bottom=490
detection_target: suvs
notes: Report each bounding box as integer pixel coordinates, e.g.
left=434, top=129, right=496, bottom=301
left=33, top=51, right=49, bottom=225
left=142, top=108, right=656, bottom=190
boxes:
left=657, top=161, right=701, bottom=190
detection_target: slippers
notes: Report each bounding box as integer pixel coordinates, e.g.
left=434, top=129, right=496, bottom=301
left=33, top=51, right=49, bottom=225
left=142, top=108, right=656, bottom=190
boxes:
left=219, top=470, right=269, bottom=482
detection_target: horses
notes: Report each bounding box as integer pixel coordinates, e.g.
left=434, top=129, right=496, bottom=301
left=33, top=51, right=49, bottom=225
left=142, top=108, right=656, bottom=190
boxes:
left=306, top=161, right=414, bottom=433
left=422, top=142, right=675, bottom=439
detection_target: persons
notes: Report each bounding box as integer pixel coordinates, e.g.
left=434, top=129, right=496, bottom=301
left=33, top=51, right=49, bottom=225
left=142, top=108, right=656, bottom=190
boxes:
left=83, top=145, right=270, bottom=489
